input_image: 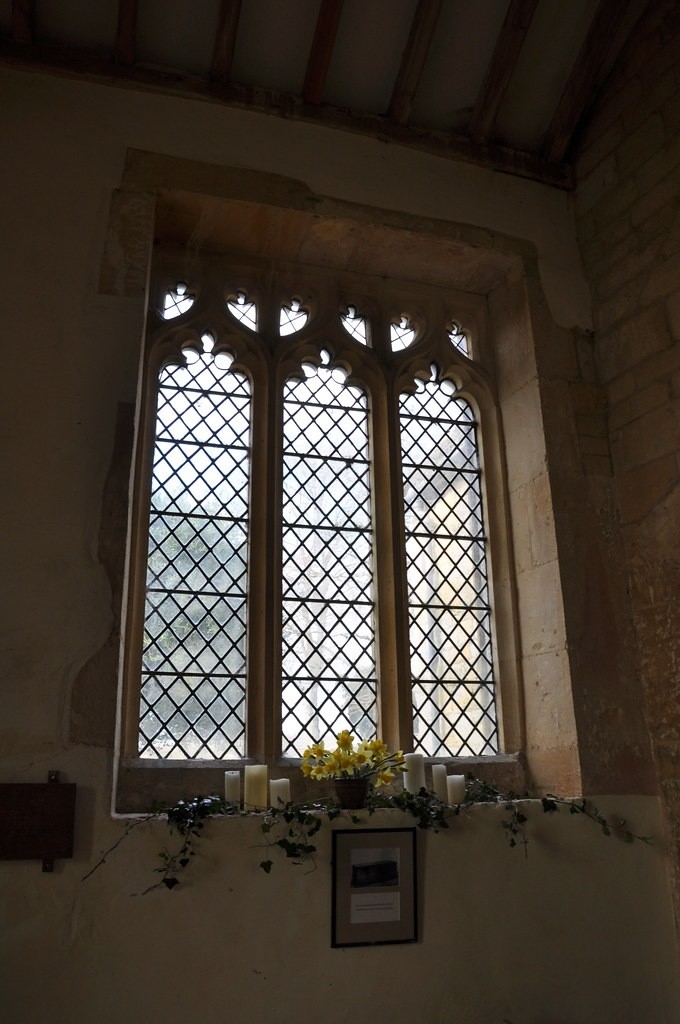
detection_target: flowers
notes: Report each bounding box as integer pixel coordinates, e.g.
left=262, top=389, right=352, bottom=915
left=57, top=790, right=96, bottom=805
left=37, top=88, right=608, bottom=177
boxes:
left=299, top=731, right=410, bottom=787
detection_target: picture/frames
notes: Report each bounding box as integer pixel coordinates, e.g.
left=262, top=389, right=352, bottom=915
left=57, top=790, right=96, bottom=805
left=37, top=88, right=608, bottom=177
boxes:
left=331, top=827, right=418, bottom=947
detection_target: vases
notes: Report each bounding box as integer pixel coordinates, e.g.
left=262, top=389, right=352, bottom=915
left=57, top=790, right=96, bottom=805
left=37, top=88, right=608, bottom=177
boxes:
left=334, top=778, right=370, bottom=809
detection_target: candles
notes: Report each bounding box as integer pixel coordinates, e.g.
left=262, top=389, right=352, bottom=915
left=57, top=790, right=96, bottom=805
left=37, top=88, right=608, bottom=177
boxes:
left=269, top=777, right=292, bottom=813
left=225, top=771, right=241, bottom=811
left=244, top=765, right=268, bottom=811
left=431, top=764, right=448, bottom=803
left=402, top=753, right=427, bottom=800
left=446, top=775, right=466, bottom=803
